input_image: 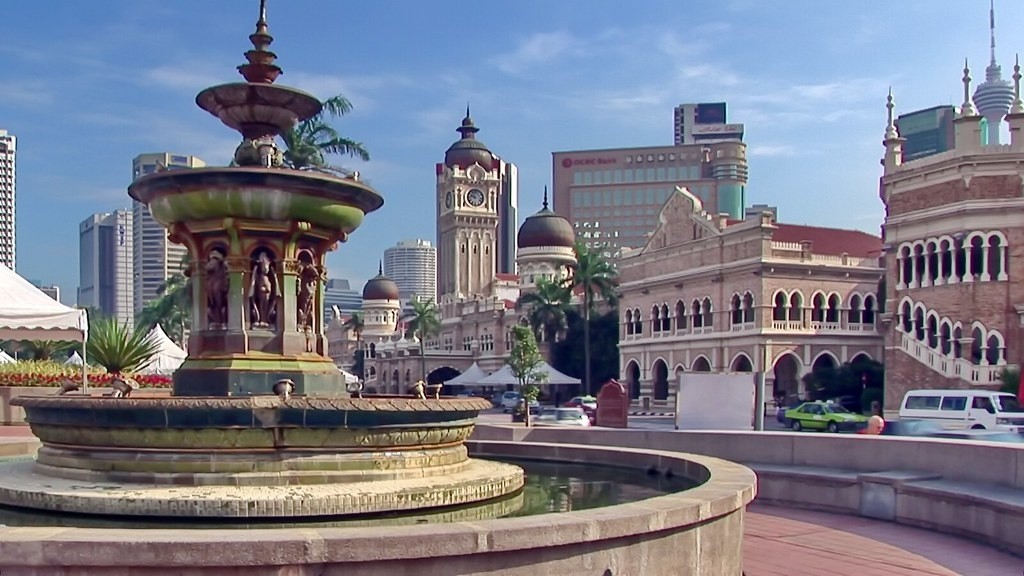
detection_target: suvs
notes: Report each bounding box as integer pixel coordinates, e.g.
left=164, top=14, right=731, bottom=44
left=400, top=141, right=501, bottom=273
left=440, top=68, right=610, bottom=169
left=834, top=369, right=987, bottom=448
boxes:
left=459, top=390, right=540, bottom=414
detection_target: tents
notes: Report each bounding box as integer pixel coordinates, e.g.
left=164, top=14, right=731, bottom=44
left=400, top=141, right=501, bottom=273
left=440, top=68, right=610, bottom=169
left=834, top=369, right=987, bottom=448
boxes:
left=0, top=262, right=87, bottom=395
left=442, top=359, right=581, bottom=400
left=123, top=323, right=188, bottom=374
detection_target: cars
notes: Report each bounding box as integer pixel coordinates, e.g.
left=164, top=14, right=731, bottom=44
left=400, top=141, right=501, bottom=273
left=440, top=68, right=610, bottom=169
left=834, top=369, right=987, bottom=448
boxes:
left=564, top=395, right=598, bottom=419
left=777, top=400, right=869, bottom=433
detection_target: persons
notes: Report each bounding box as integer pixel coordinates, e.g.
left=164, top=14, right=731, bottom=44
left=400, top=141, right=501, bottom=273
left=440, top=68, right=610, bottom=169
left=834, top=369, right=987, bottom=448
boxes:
left=856, top=416, right=884, bottom=435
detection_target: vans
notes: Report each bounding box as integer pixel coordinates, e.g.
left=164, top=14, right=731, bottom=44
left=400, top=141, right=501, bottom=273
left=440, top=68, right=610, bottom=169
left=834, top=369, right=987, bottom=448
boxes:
left=899, top=389, right=1024, bottom=433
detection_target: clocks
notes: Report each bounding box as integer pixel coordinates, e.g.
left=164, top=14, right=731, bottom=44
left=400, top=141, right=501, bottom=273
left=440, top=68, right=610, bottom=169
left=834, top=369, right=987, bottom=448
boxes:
left=467, top=189, right=484, bottom=206
